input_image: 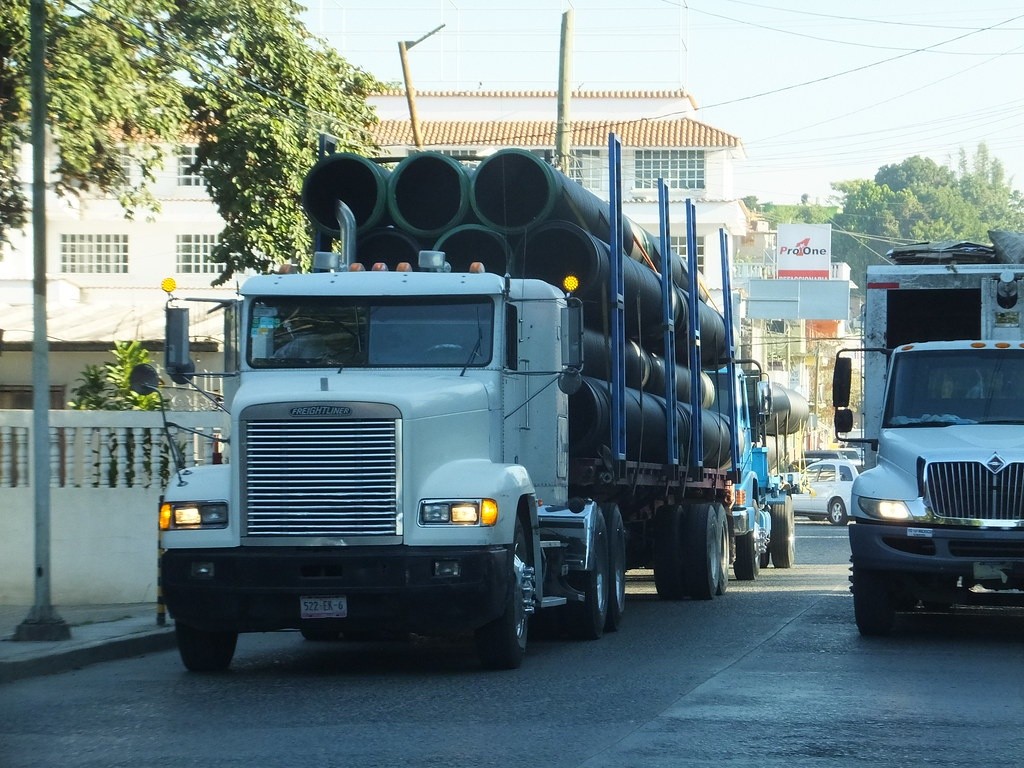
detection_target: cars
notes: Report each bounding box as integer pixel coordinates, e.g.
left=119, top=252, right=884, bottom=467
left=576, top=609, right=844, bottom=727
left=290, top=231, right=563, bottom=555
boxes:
left=782, top=428, right=865, bottom=526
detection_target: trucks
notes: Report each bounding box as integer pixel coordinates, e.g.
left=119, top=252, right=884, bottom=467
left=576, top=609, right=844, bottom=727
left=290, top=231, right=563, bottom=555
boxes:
left=833, top=263, right=1024, bottom=636
left=130, top=133, right=795, bottom=671
